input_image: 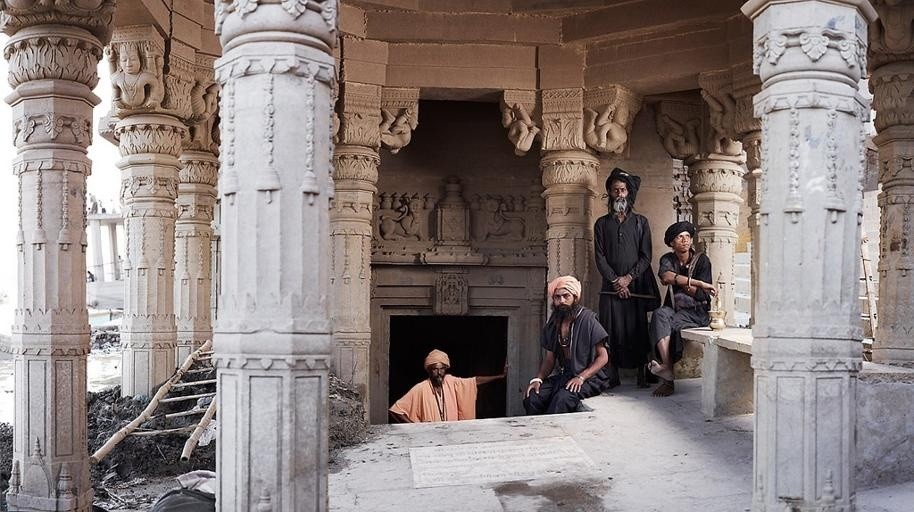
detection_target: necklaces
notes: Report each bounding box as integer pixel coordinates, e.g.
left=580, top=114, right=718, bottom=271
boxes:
left=430, top=379, right=445, bottom=421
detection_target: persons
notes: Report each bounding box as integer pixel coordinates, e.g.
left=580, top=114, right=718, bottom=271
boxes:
left=100, top=43, right=221, bottom=130
left=594, top=167, right=660, bottom=389
left=499, top=89, right=744, bottom=160
left=522, top=274, right=609, bottom=414
left=647, top=220, right=719, bottom=396
left=376, top=102, right=418, bottom=156
left=387, top=348, right=507, bottom=424
left=866, top=0, right=913, bottom=56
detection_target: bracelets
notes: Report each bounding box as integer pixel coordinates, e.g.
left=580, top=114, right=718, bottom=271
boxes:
left=529, top=377, right=543, bottom=385
left=577, top=374, right=585, bottom=383
left=688, top=277, right=690, bottom=291
left=673, top=274, right=678, bottom=286
